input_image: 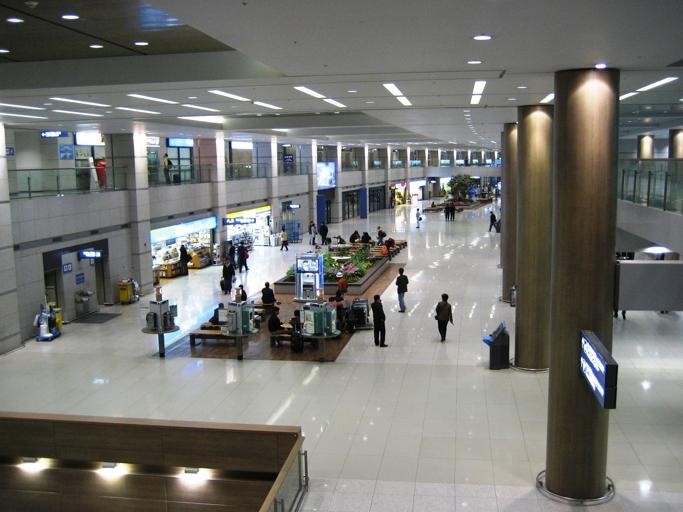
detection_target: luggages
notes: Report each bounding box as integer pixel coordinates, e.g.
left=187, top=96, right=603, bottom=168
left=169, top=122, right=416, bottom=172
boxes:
left=291, top=322, right=304, bottom=354
left=173, top=168, right=181, bottom=184
left=219, top=276, right=226, bottom=290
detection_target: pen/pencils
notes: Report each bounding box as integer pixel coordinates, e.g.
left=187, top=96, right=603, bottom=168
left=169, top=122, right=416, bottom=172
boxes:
left=510, top=286, right=515, bottom=307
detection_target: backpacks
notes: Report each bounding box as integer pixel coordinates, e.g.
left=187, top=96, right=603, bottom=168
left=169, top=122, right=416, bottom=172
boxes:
left=167, top=159, right=172, bottom=170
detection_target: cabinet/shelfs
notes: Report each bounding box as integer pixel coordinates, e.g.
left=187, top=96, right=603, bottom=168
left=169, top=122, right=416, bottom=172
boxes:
left=152, top=229, right=214, bottom=279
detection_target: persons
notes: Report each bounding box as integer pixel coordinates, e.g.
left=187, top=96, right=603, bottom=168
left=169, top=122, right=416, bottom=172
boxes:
left=279, top=229, right=288, bottom=251
left=261, top=281, right=282, bottom=305
left=336, top=270, right=348, bottom=294
left=236, top=241, right=251, bottom=273
left=222, top=258, right=235, bottom=294
left=443, top=203, right=449, bottom=223
left=449, top=202, right=455, bottom=222
left=228, top=241, right=237, bottom=272
left=414, top=208, right=421, bottom=229
left=239, top=284, right=247, bottom=301
left=308, top=220, right=394, bottom=252
left=290, top=310, right=304, bottom=332
left=334, top=291, right=345, bottom=301
left=268, top=306, right=285, bottom=335
left=371, top=295, right=389, bottom=348
left=487, top=211, right=497, bottom=233
left=160, top=153, right=174, bottom=185
left=394, top=267, right=409, bottom=312
left=432, top=292, right=454, bottom=343
left=179, top=246, right=188, bottom=275
left=209, top=302, right=227, bottom=326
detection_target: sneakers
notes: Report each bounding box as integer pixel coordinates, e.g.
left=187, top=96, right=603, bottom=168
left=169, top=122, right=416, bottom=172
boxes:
left=380, top=343, right=388, bottom=347
left=276, top=301, right=281, bottom=305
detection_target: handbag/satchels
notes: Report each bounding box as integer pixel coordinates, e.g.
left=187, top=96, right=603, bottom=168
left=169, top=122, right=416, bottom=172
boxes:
left=242, top=250, right=249, bottom=258
left=380, top=231, right=386, bottom=237
left=183, top=253, right=192, bottom=263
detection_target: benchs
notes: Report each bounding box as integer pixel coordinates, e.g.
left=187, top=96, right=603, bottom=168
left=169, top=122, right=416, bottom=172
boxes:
left=422, top=205, right=463, bottom=214
left=188, top=294, right=298, bottom=346
left=329, top=237, right=408, bottom=260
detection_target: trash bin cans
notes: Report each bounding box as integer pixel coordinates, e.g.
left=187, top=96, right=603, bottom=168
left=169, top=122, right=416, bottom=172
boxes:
left=116, top=279, right=134, bottom=305
left=482, top=322, right=509, bottom=370
left=75, top=291, right=98, bottom=315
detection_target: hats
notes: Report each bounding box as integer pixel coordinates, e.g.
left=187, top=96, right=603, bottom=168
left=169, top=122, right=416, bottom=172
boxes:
left=336, top=272, right=343, bottom=278
